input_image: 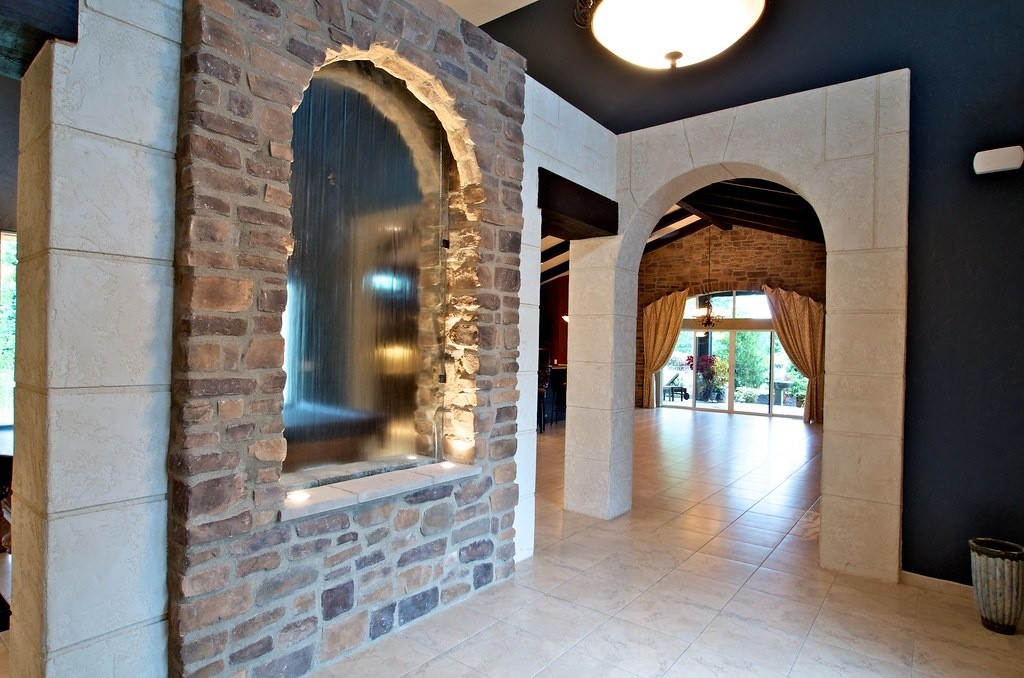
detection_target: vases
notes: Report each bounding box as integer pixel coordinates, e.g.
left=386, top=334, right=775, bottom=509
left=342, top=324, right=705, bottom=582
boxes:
left=968, top=537, right=1024, bottom=635
left=707, top=392, right=717, bottom=402
left=715, top=388, right=725, bottom=403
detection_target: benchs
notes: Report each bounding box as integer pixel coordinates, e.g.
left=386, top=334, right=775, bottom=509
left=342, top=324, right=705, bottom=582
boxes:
left=663, top=386, right=683, bottom=402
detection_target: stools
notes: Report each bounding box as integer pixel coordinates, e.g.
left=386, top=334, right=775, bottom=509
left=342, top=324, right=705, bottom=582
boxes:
left=550, top=382, right=566, bottom=426
left=536, top=387, right=547, bottom=433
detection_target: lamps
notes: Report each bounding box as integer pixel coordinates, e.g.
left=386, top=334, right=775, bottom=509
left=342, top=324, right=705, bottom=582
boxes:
left=691, top=226, right=726, bottom=329
left=572, top=0, right=766, bottom=71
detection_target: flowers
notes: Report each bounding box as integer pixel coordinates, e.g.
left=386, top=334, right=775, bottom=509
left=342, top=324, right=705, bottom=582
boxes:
left=711, top=357, right=729, bottom=388
left=686, top=355, right=718, bottom=400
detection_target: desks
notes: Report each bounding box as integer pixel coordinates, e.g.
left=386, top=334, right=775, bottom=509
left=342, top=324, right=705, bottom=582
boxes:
left=283, top=400, right=388, bottom=473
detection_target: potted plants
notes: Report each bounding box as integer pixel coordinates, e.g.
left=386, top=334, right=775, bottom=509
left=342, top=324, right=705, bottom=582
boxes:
left=796, top=394, right=805, bottom=408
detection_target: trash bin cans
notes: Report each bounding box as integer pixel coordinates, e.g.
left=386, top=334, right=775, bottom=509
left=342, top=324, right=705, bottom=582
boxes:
left=968, top=537, right=1024, bottom=635
